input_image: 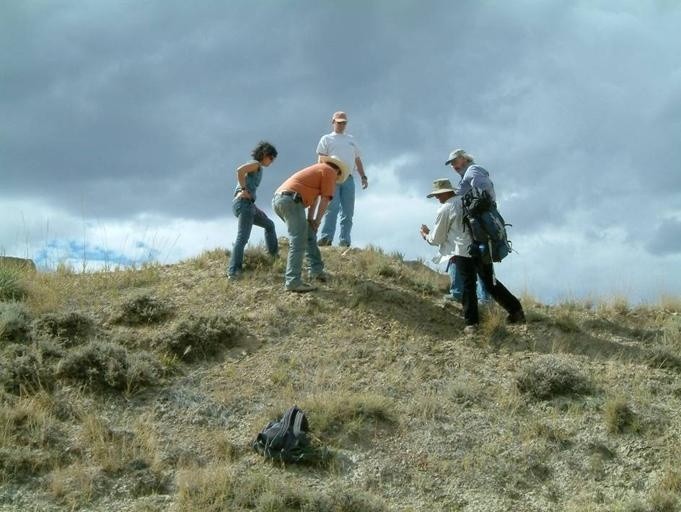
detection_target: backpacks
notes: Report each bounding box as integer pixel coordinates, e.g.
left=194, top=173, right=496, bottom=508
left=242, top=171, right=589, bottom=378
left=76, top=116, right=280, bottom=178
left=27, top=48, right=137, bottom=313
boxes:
left=461, top=187, right=512, bottom=264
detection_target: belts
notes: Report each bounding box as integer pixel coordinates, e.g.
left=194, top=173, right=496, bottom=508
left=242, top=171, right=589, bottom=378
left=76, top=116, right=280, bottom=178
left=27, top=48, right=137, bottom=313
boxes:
left=282, top=191, right=303, bottom=203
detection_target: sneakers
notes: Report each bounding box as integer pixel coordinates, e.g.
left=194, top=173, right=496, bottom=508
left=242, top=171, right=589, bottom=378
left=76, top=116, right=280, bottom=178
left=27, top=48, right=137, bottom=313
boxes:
left=285, top=281, right=317, bottom=291
left=477, top=298, right=490, bottom=307
left=317, top=239, right=332, bottom=246
left=504, top=311, right=527, bottom=324
left=227, top=273, right=245, bottom=284
left=443, top=294, right=463, bottom=302
left=463, top=325, right=478, bottom=337
left=314, top=275, right=327, bottom=285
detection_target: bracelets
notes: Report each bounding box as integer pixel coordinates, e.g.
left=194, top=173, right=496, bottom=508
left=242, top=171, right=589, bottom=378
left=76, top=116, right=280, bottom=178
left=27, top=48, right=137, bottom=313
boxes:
left=241, top=187, right=247, bottom=191
left=423, top=234, right=427, bottom=240
left=361, top=175, right=367, bottom=180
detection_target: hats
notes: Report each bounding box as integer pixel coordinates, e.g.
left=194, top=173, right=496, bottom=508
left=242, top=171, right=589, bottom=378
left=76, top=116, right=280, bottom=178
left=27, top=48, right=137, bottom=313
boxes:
left=426, top=178, right=458, bottom=198
left=332, top=111, right=348, bottom=122
left=319, top=155, right=350, bottom=184
left=445, top=148, right=466, bottom=166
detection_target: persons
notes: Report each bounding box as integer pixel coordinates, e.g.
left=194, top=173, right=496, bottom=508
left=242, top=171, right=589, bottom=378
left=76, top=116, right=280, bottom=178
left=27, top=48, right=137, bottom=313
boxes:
left=419, top=178, right=527, bottom=335
left=315, top=111, right=369, bottom=246
left=271, top=155, right=350, bottom=292
left=227, top=141, right=282, bottom=280
left=443, top=149, right=496, bottom=304
